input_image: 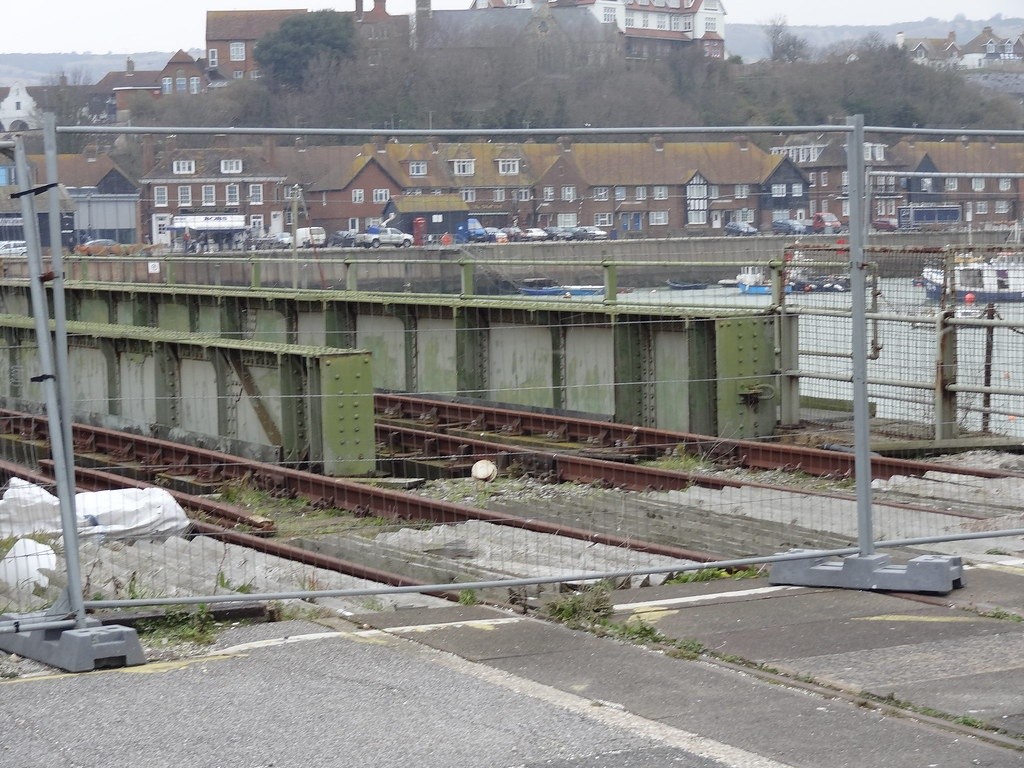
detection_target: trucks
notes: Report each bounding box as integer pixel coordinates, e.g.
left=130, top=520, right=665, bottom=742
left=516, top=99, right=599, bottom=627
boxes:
left=799, top=212, right=842, bottom=233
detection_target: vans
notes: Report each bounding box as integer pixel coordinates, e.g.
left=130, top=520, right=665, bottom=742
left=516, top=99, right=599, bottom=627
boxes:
left=0, top=240, right=28, bottom=256
left=469, top=218, right=486, bottom=242
left=296, top=228, right=326, bottom=247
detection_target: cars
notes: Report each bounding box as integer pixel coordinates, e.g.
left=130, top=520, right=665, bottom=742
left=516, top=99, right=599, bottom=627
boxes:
left=484, top=227, right=508, bottom=243
left=772, top=219, right=807, bottom=235
left=501, top=228, right=527, bottom=242
left=330, top=230, right=357, bottom=247
left=544, top=227, right=574, bottom=241
left=565, top=226, right=608, bottom=239
left=871, top=216, right=899, bottom=232
left=723, top=221, right=757, bottom=236
left=84, top=238, right=119, bottom=257
left=522, top=228, right=549, bottom=241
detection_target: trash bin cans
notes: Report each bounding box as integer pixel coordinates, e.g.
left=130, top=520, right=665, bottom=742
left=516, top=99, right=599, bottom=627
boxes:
left=610, top=229, right=617, bottom=240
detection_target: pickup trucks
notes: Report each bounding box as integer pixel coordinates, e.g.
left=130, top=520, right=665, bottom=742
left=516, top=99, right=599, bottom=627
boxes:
left=353, top=224, right=415, bottom=248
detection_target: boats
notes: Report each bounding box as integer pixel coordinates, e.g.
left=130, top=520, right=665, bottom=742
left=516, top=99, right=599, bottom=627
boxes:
left=735, top=246, right=878, bottom=295
left=921, top=221, right=1024, bottom=304
left=518, top=278, right=606, bottom=294
left=665, top=279, right=711, bottom=289
left=718, top=278, right=740, bottom=288
